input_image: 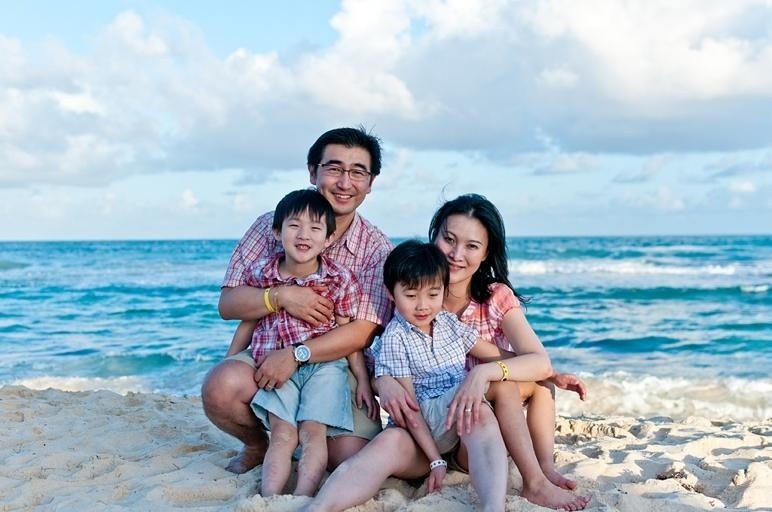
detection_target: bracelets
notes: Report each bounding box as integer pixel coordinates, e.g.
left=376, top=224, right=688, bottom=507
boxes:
left=264, top=288, right=275, bottom=313
left=496, top=359, right=509, bottom=382
left=429, top=460, right=447, bottom=470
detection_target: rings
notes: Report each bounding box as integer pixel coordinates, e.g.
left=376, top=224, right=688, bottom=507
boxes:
left=465, top=409, right=471, bottom=411
left=267, top=384, right=273, bottom=389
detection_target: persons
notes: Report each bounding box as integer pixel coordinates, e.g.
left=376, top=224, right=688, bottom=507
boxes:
left=304, top=192, right=553, bottom=511
left=363, top=239, right=592, bottom=512
left=201, top=128, right=394, bottom=472
left=226, top=188, right=380, bottom=497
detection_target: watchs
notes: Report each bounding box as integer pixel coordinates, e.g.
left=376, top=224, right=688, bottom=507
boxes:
left=292, top=342, right=311, bottom=366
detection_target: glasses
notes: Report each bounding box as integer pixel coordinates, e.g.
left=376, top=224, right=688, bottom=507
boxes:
left=317, top=163, right=373, bottom=181
